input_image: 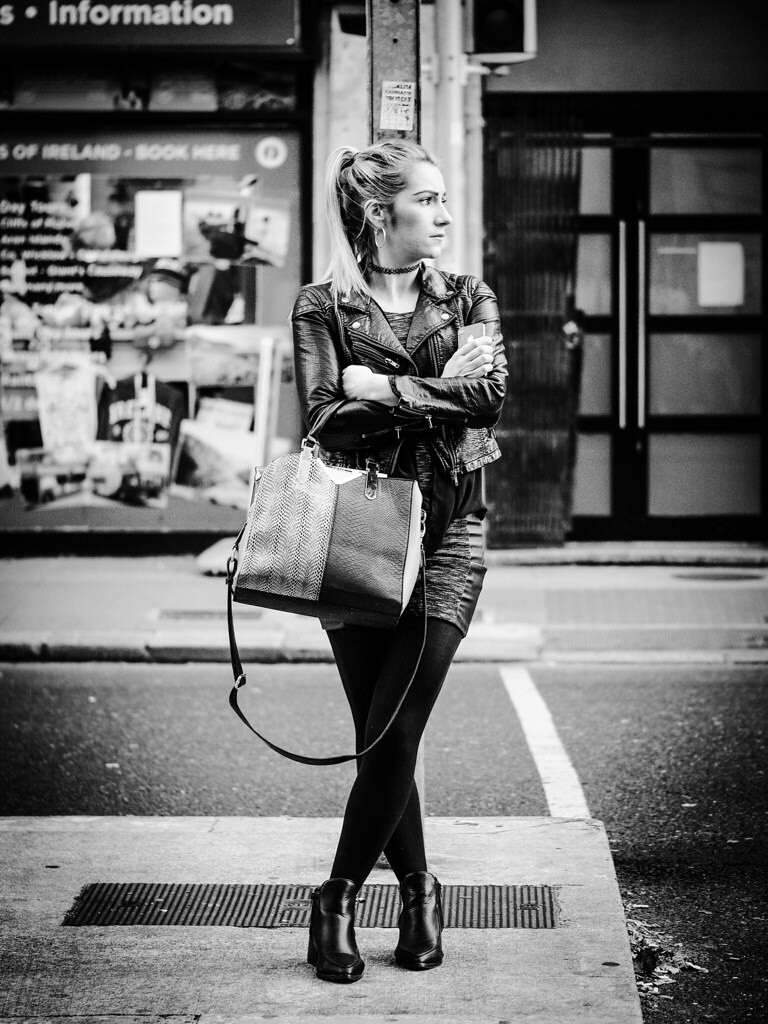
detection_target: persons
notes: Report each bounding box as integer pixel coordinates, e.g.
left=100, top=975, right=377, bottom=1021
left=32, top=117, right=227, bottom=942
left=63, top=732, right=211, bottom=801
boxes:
left=291, top=140, right=508, bottom=985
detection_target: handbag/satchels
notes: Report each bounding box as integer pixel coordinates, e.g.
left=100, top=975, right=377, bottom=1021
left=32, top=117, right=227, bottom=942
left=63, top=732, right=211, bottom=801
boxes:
left=233, top=398, right=425, bottom=612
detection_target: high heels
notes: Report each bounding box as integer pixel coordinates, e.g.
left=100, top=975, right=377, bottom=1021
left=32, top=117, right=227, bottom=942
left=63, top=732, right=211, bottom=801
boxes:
left=307, top=880, right=363, bottom=986
left=393, top=871, right=443, bottom=968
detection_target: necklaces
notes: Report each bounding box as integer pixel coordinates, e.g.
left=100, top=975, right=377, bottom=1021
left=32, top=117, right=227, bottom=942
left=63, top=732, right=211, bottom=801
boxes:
left=368, top=262, right=420, bottom=274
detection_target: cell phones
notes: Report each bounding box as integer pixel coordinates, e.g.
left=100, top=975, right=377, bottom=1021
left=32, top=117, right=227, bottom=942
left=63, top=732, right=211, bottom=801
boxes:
left=459, top=322, right=487, bottom=377
